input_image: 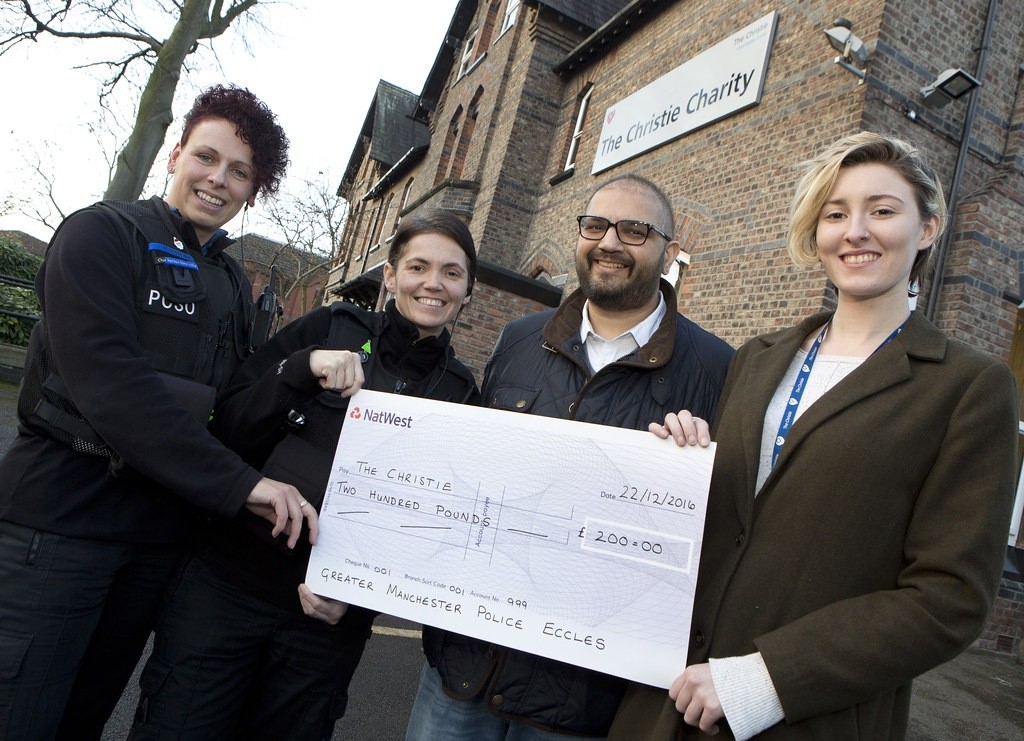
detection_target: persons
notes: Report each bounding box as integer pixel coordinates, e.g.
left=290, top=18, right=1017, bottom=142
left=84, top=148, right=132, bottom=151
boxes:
left=124, top=209, right=484, bottom=741
left=401, top=174, right=738, bottom=741
left=0, top=84, right=320, bottom=741
left=582, top=131, right=1020, bottom=741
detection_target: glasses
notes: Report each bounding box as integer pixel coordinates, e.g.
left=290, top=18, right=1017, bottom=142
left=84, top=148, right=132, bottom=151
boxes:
left=577, top=214, right=672, bottom=246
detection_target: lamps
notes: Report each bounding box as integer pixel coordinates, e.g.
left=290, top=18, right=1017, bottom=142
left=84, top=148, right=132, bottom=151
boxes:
left=920, top=66, right=982, bottom=111
left=824, top=24, right=873, bottom=80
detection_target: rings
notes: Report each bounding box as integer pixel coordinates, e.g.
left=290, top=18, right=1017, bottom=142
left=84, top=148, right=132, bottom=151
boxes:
left=298, top=500, right=308, bottom=507
